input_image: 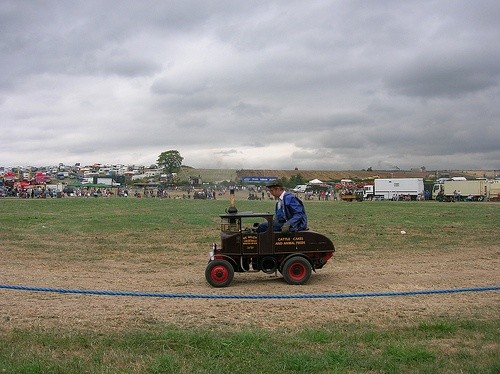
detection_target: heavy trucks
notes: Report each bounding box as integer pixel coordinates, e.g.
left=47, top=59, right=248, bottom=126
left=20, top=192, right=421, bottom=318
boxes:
left=432, top=180, right=486, bottom=202
left=363, top=177, right=424, bottom=201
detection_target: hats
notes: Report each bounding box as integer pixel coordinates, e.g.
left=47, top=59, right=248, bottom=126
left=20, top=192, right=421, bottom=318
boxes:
left=266, top=179, right=284, bottom=187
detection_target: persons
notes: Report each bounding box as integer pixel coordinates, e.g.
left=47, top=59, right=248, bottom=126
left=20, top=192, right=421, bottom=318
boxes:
left=391, top=194, right=411, bottom=201
left=0, top=186, right=226, bottom=200
left=438, top=189, right=462, bottom=203
left=305, top=187, right=353, bottom=200
left=256, top=180, right=307, bottom=234
left=425, top=190, right=430, bottom=200
left=248, top=192, right=258, bottom=200
left=498, top=193, right=500, bottom=202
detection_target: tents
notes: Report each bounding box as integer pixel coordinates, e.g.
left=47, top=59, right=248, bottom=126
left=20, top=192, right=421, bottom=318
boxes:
left=309, top=178, right=323, bottom=186
